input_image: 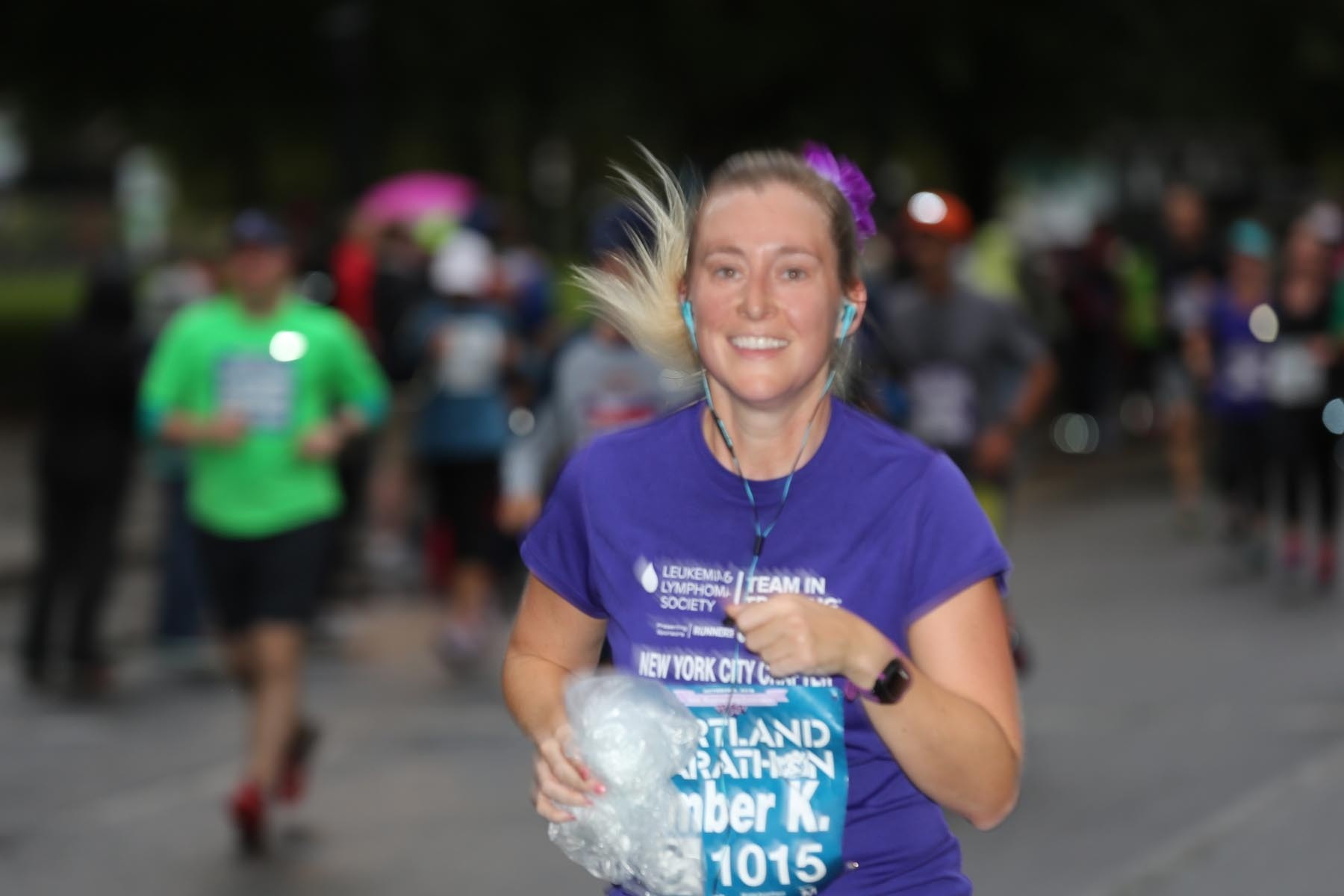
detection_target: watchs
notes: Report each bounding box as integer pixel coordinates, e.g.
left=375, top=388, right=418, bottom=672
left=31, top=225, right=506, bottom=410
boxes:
left=843, top=644, right=914, bottom=702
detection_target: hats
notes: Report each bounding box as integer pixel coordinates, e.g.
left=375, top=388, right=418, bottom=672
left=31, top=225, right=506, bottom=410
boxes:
left=221, top=210, right=286, bottom=251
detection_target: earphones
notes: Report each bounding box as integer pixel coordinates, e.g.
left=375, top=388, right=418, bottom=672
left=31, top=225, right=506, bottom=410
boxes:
left=681, top=300, right=699, bottom=350
left=840, top=301, right=857, bottom=337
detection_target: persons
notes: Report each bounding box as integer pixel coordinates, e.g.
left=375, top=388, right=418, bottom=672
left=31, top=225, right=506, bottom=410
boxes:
left=498, top=140, right=1025, bottom=895
left=0, top=164, right=1343, bottom=850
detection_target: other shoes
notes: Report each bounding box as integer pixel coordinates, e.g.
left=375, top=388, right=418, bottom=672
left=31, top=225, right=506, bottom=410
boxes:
left=273, top=718, right=320, bottom=799
left=229, top=786, right=269, bottom=862
